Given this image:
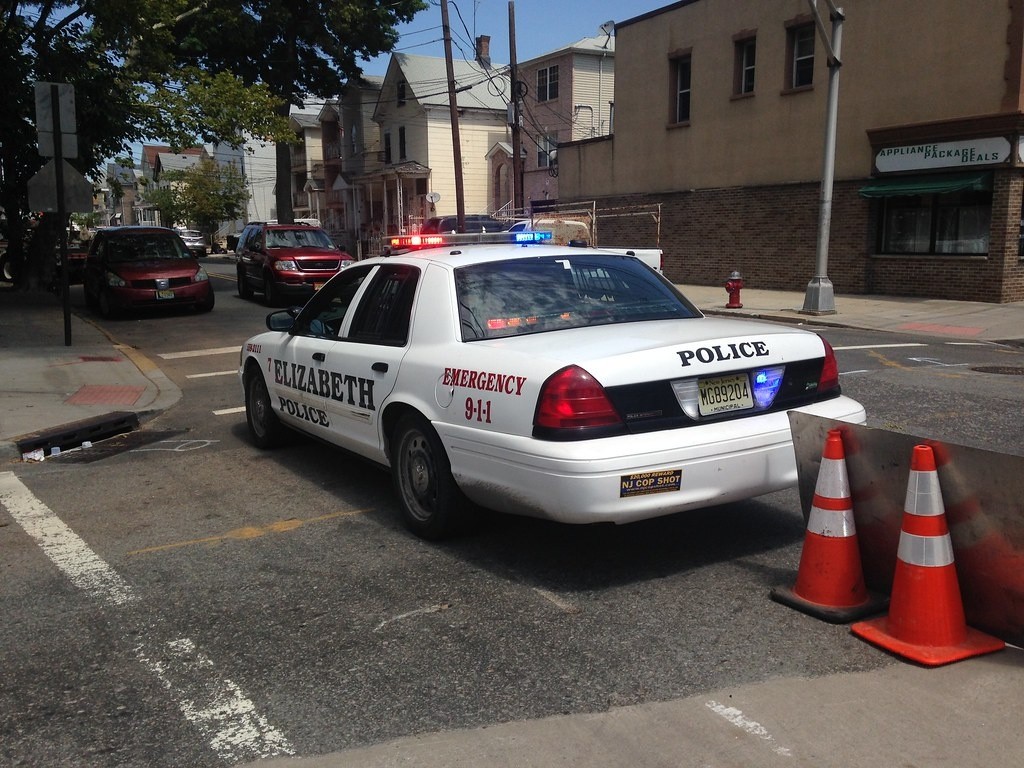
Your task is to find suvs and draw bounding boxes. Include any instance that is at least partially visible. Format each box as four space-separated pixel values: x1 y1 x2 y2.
419 214 511 234
236 221 355 304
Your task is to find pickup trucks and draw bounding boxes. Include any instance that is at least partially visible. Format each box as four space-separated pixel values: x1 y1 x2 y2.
506 200 663 278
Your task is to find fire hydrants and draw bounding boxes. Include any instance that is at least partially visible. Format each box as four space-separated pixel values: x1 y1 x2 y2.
724 270 745 310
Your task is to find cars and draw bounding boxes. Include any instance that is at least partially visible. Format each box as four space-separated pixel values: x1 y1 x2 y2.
235 223 869 543
0 222 215 321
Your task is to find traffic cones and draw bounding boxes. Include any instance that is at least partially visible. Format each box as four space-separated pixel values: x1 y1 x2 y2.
926 440 1023 637
818 423 899 576
768 428 892 623
852 445 1008 668
